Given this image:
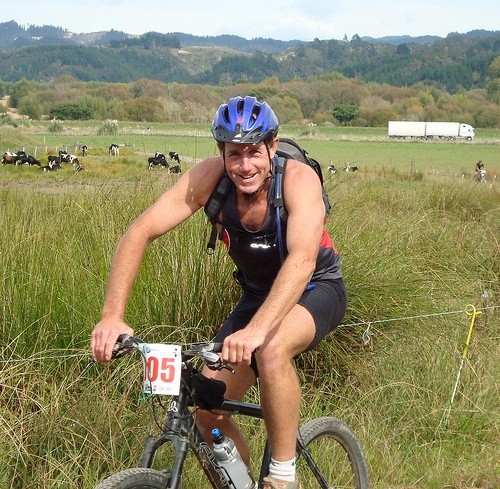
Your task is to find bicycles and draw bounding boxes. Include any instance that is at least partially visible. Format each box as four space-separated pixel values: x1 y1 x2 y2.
473 169 486 183
90 329 371 489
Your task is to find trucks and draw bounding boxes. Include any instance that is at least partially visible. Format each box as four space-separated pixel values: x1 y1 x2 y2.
387 120 476 142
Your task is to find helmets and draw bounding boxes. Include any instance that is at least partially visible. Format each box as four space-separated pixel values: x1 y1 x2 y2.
210 95 280 145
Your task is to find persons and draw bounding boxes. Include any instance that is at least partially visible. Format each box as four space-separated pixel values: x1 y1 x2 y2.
476 160 484 177
91 96 348 489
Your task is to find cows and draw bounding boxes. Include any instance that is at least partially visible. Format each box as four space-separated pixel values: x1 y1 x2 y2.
80 145 88 156
1 150 81 172
327 160 358 174
108 143 120 156
147 151 181 173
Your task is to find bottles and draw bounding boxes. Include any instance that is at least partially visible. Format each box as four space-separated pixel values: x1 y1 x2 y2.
211 427 258 489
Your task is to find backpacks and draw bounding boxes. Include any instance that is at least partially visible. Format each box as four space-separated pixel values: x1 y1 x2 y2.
203 137 332 292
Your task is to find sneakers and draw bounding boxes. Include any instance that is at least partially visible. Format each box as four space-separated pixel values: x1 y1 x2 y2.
261 474 303 489
